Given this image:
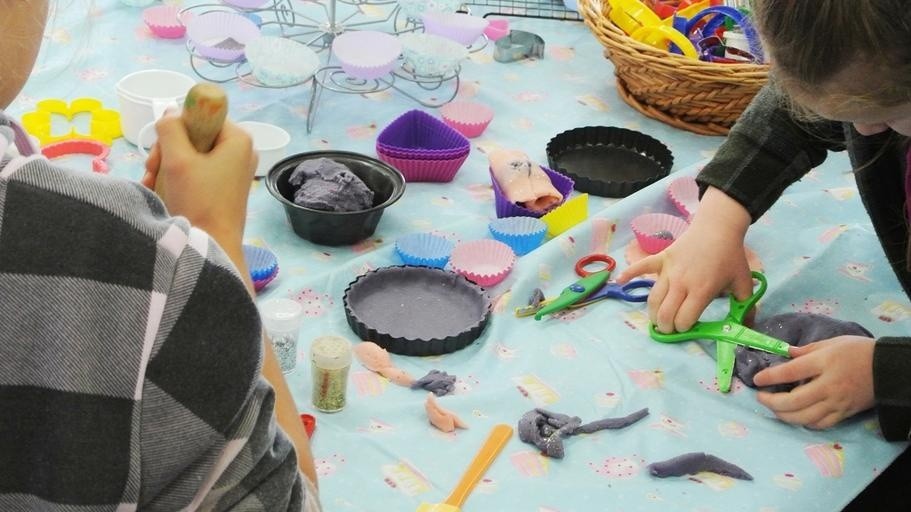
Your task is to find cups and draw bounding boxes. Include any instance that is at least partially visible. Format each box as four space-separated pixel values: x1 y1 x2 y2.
236 122 291 179
313 336 352 417
113 69 196 151
261 297 301 375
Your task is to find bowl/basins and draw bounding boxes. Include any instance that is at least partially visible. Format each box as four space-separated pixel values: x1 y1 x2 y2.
267 149 403 246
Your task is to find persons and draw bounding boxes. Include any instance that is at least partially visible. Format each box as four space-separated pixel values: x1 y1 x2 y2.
1 1 323 512
608 1 910 441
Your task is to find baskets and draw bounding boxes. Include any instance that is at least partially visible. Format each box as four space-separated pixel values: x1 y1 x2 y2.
578 0 773 138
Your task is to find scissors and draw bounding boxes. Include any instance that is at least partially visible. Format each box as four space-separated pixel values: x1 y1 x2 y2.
647 269 793 392
514 254 655 321
